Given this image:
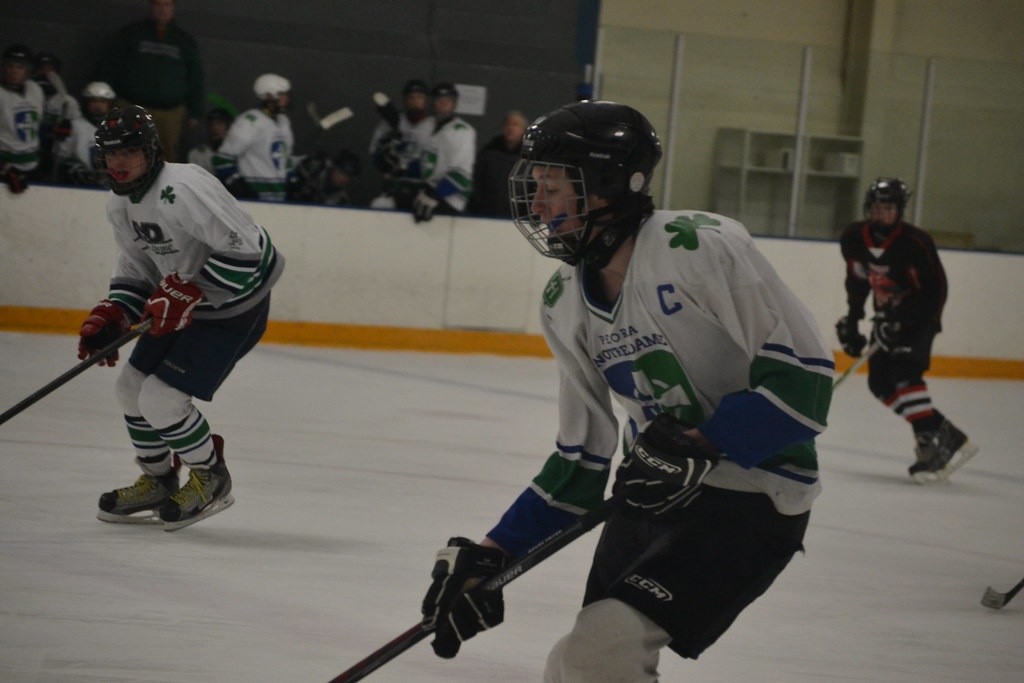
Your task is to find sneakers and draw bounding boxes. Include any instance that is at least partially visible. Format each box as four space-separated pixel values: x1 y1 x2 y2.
159 434 235 532
908 426 978 482
95 451 183 524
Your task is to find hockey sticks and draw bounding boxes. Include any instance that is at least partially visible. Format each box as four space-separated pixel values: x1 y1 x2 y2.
981 578 1024 610
832 340 882 391
0 316 154 428
329 495 621 683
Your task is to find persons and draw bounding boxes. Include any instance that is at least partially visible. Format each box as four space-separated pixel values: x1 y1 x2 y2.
97 0 204 127
425 99 836 683
835 177 980 485
77 105 286 532
0 43 539 225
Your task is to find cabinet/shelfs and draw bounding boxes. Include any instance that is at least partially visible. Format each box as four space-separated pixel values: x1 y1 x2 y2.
710 126 867 240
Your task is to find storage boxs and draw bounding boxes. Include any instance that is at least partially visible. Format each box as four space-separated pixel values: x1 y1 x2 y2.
823 152 859 175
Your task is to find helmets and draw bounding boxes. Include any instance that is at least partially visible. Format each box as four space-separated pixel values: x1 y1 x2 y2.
254 74 291 113
509 100 664 259
863 177 908 230
2 46 30 86
206 108 231 141
433 84 457 116
93 104 164 195
82 81 116 120
402 80 430 114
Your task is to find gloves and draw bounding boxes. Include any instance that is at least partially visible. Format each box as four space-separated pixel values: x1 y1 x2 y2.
78 299 131 366
836 318 866 358
141 271 202 336
612 415 721 523
421 537 504 659
413 187 436 223
2 166 30 194
